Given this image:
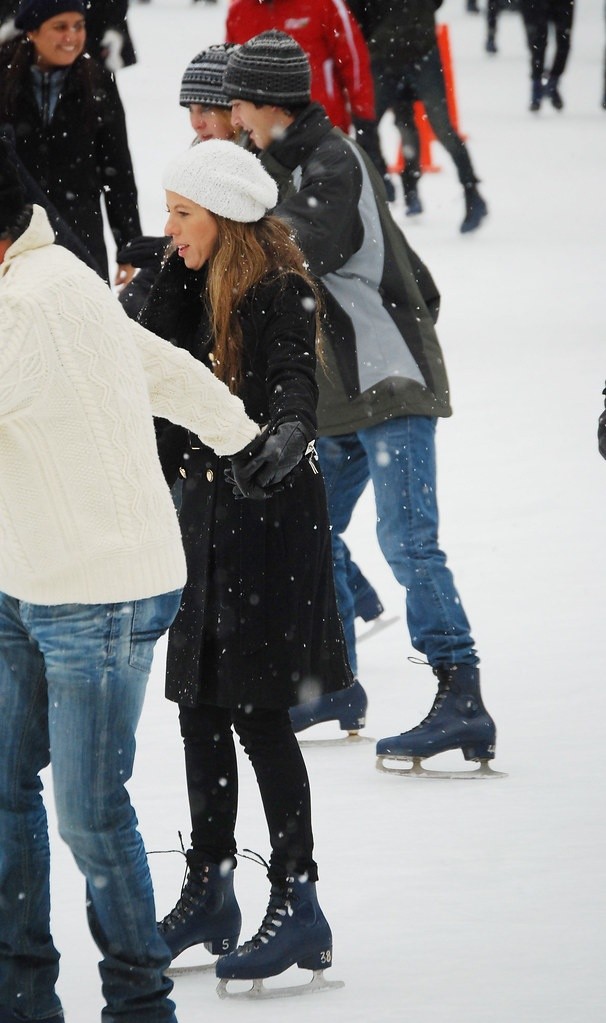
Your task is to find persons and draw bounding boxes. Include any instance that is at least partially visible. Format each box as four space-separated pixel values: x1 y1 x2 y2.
0 0 143 293
132 137 345 1003
115 30 509 780
345 0 575 234
225 0 377 136
0 137 295 1023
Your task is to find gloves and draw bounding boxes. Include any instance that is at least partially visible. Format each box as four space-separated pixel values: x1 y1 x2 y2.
223 422 310 499
118 236 171 268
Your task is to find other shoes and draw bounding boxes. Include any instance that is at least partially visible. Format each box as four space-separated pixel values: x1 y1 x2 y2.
529 84 545 110
485 40 498 53
545 81 563 109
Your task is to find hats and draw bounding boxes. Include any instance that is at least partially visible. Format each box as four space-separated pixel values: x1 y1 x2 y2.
179 41 237 110
221 30 312 103
15 0 91 30
164 138 277 222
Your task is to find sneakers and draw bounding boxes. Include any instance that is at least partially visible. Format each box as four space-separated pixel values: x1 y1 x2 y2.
141 831 242 960
286 679 368 742
214 848 346 999
458 184 489 233
376 656 510 781
348 571 400 642
400 172 423 216
